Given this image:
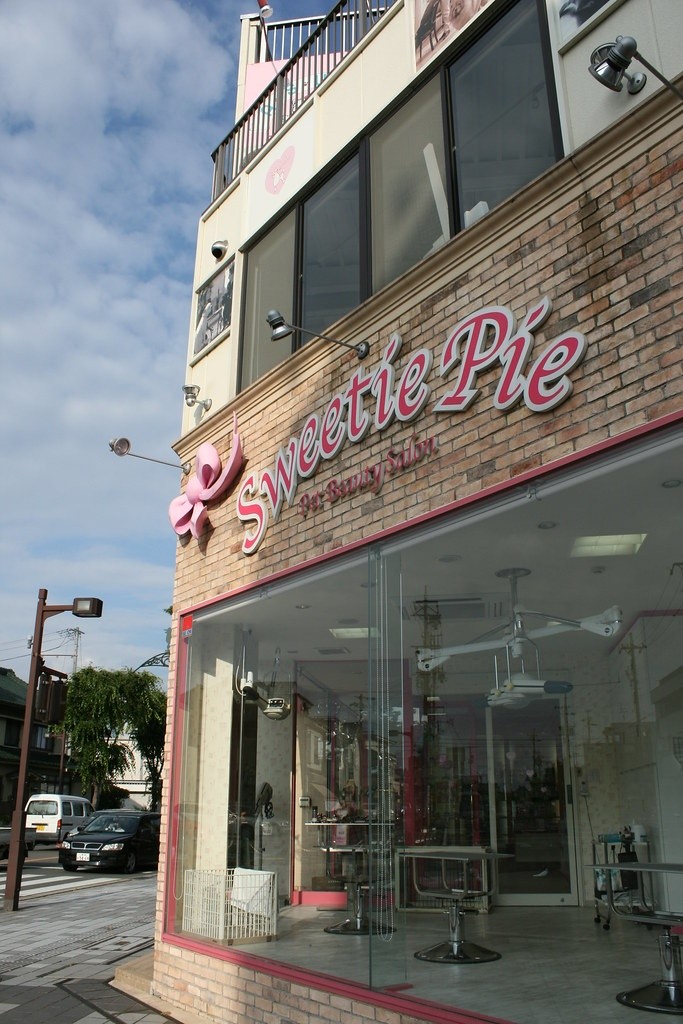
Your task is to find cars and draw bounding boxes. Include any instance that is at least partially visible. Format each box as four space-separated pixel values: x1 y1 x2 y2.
59 808 161 874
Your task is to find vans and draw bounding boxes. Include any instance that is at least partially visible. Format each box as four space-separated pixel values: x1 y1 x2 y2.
24 794 97 851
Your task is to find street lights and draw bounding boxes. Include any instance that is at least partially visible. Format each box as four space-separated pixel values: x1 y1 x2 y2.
3 588 103 912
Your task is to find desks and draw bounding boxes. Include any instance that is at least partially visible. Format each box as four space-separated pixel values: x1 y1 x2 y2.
313 844 399 936
582 861 683 1016
397 849 517 965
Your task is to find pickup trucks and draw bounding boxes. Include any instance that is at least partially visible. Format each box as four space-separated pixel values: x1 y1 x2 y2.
0 826 38 866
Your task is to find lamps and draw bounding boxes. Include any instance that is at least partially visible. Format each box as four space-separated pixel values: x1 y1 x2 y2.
266 310 369 360
108 437 191 475
588 34 683 101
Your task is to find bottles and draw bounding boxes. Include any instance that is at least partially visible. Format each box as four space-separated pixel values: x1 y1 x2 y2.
312 806 318 823
